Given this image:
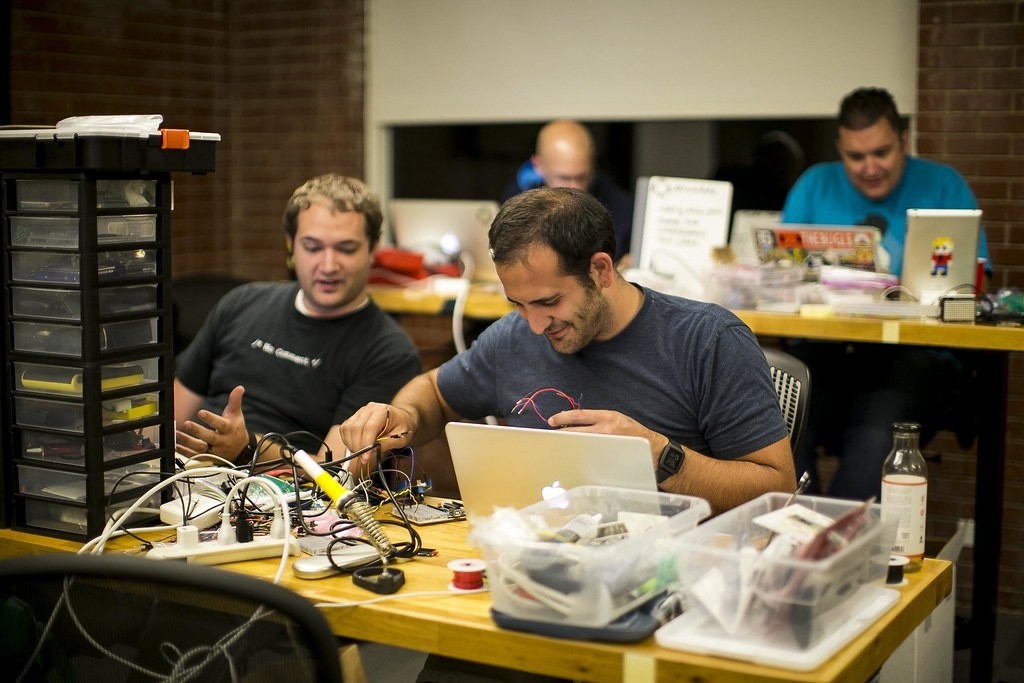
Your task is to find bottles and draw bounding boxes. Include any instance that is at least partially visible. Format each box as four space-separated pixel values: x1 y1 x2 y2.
878 423 928 572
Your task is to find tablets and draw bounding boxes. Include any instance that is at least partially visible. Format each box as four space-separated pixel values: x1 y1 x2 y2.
898 207 984 301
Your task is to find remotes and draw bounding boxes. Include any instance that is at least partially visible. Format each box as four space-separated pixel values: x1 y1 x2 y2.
292 543 381 581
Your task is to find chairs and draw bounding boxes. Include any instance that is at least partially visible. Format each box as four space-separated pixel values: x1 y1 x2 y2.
173 271 253 356
0 552 347 683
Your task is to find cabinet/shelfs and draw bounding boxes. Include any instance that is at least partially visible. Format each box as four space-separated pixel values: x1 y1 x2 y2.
0 174 175 544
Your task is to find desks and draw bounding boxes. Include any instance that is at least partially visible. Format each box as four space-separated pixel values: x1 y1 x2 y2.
369 268 1024 683
0 451 956 683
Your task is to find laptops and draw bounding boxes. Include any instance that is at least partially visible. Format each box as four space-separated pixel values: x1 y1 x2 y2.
389 198 506 284
442 422 659 531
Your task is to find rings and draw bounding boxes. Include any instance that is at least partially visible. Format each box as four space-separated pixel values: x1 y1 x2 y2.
202 442 212 454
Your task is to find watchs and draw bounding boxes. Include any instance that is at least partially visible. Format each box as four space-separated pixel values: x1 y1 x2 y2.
653 437 686 484
226 429 257 469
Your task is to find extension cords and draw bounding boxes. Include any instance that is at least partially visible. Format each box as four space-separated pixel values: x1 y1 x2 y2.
147 533 294 566
161 485 226 531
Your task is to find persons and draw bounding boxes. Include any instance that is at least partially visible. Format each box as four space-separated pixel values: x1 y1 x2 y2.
434 122 637 365
340 186 797 682
1 175 423 683
780 88 992 508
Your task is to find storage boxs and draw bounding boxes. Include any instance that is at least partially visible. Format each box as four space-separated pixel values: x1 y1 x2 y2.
670 491 900 654
0 124 222 178
469 484 711 645
